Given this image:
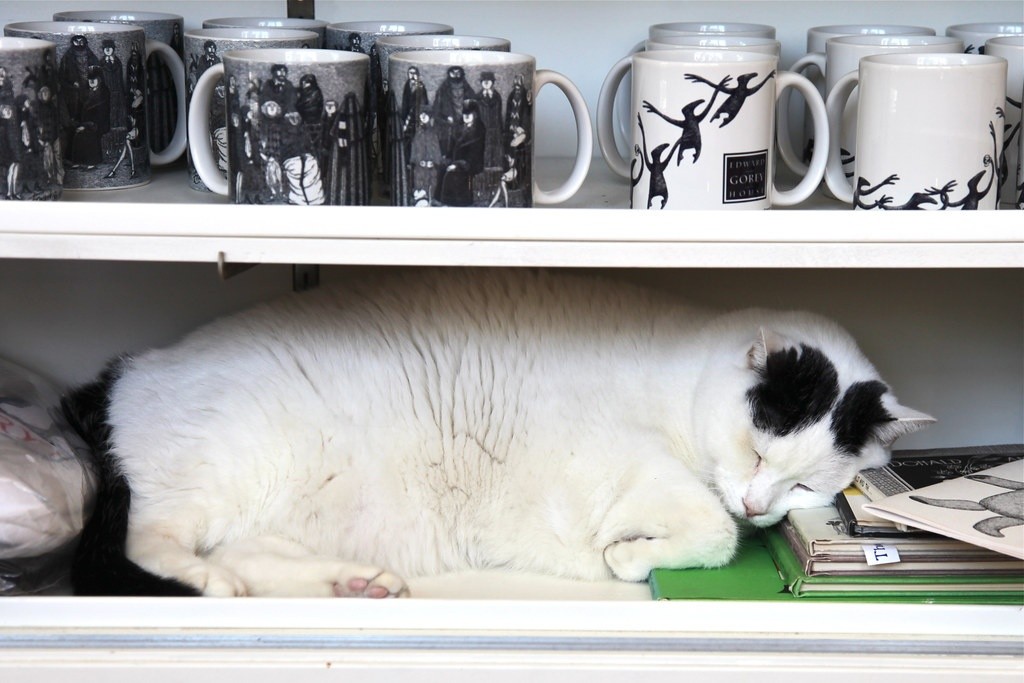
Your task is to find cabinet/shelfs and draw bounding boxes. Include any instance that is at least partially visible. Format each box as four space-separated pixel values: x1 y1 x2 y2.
0 172 1024 683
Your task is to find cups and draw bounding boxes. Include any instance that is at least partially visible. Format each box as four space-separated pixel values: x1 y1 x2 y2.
596 21 1024 212
0 9 594 208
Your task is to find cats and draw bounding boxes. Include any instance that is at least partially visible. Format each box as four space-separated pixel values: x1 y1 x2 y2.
0 266 940 599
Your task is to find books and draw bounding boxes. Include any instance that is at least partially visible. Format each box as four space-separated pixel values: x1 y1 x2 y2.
763 444 1023 601
